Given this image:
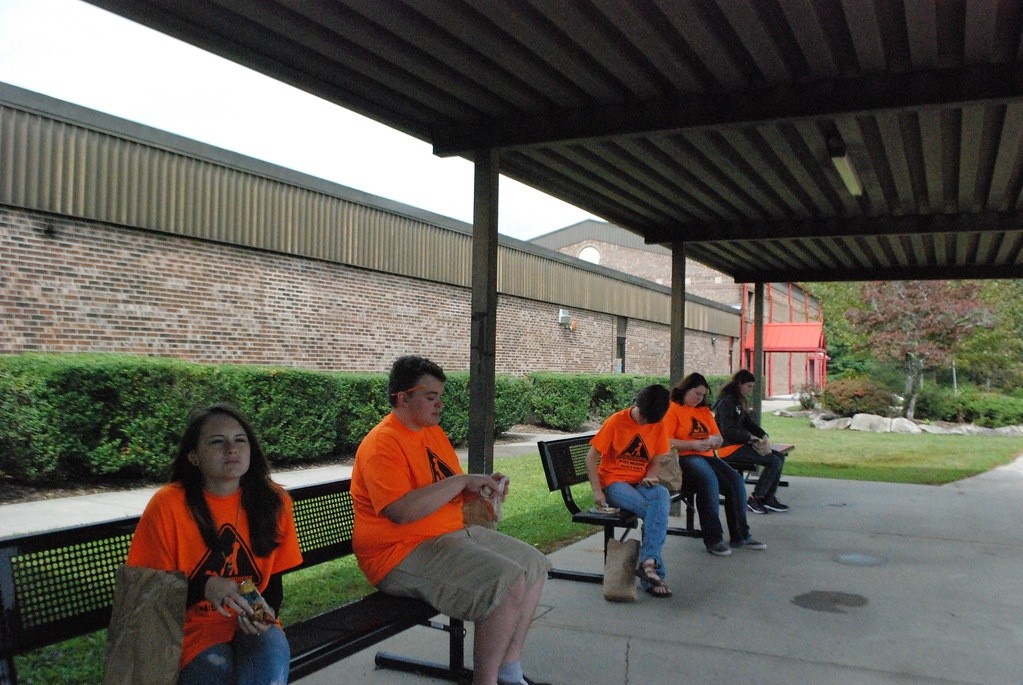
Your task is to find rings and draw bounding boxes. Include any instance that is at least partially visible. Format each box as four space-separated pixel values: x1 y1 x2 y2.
222 595 230 603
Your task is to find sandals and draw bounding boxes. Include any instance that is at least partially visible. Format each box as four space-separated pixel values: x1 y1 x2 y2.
635 563 661 586
640 579 672 597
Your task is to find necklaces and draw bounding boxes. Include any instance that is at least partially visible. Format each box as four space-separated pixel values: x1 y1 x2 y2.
200 485 244 573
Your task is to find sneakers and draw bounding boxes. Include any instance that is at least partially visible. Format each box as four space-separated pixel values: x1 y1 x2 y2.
707 541 731 555
747 496 768 513
730 537 766 549
763 499 789 511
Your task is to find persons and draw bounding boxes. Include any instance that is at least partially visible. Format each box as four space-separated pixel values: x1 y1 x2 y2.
665 372 767 556
584 384 673 597
712 369 790 514
124 403 304 685
349 354 553 685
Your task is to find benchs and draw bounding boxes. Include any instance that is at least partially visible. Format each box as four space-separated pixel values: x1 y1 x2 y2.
0 478 551 685
538 435 796 584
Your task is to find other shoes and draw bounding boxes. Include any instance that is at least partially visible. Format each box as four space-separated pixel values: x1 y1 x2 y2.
499 675 549 685
